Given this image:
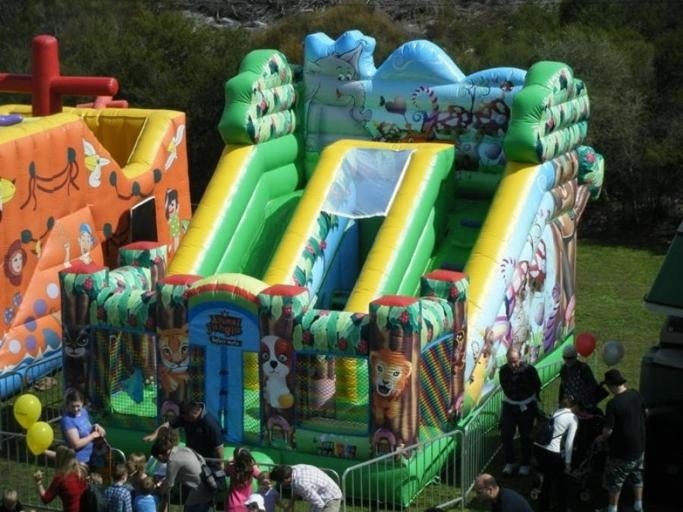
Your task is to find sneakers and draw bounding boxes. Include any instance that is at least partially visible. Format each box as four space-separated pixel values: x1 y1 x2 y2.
595 506 608 512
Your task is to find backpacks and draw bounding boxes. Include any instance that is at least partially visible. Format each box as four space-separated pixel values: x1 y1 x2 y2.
528 414 554 446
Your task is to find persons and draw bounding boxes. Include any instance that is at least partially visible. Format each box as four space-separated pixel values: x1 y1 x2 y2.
142 401 342 512
476 345 652 512
33 392 157 511
1 485 24 511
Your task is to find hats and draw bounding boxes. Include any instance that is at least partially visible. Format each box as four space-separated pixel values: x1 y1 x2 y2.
599 369 627 387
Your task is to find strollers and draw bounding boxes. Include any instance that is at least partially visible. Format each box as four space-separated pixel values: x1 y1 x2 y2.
518 406 593 504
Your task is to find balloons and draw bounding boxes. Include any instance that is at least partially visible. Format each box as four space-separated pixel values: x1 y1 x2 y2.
603 340 624 365
27 421 54 455
576 333 595 357
13 394 42 429
